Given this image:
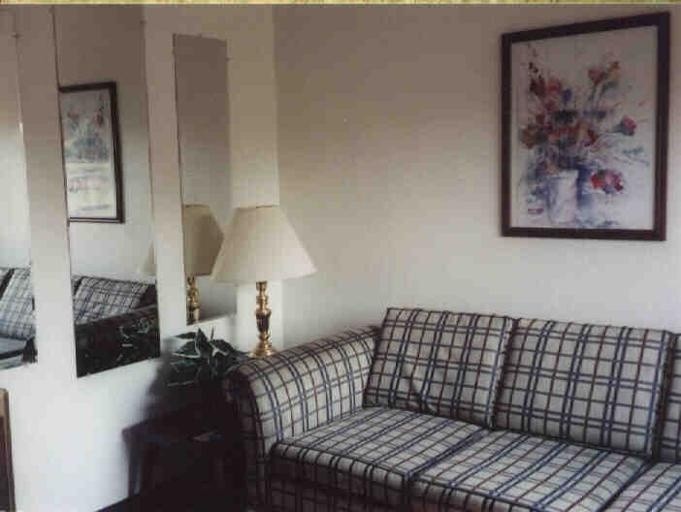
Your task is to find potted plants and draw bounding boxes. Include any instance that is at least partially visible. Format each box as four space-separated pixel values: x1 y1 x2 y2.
168 327 247 418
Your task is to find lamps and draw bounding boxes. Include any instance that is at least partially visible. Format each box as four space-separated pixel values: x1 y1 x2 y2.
135 198 219 327
212 205 315 357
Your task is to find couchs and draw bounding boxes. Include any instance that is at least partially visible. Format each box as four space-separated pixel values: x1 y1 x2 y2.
0 267 158 378
224 307 679 512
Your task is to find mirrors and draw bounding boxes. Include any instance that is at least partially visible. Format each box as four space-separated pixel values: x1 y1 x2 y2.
52 5 163 377
172 32 238 324
0 10 39 374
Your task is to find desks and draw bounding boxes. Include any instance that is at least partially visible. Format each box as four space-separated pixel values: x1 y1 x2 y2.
120 405 243 511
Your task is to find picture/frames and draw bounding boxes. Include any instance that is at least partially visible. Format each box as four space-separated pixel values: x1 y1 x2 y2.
498 12 671 242
57 81 125 226
